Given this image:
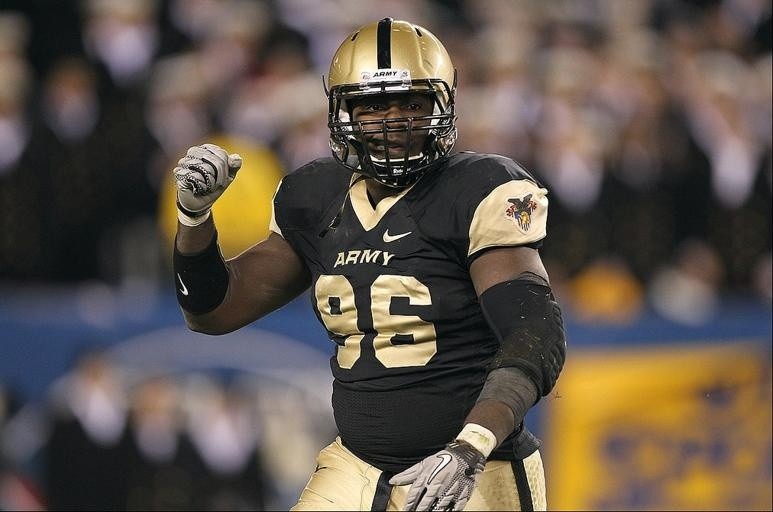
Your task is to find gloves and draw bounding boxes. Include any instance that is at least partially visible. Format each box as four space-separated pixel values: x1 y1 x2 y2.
388 423 497 512
172 143 242 227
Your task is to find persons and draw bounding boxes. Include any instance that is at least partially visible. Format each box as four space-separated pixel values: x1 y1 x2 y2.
171 16 568 511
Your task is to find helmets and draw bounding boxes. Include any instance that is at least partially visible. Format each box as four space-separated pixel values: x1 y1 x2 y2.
321 18 457 190
